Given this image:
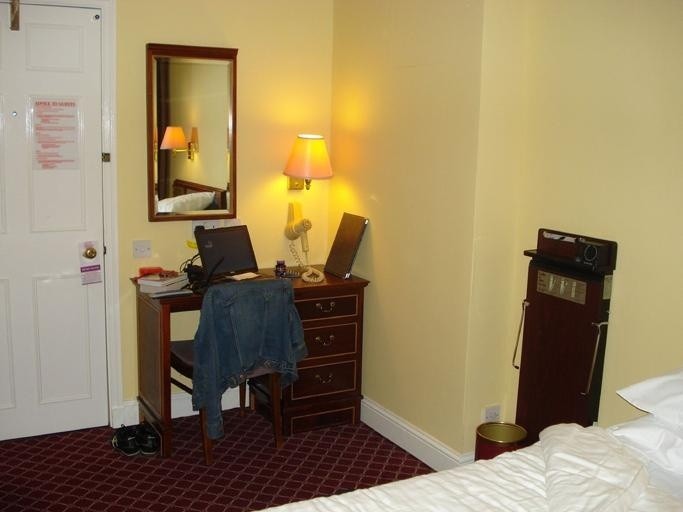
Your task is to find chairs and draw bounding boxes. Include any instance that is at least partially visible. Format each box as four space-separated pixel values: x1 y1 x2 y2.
171 279 291 465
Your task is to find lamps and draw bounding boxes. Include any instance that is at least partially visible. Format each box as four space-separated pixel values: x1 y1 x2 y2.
284 134 333 191
160 127 199 161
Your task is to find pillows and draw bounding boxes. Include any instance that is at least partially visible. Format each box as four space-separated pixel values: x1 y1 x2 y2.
606 414 683 475
607 371 682 424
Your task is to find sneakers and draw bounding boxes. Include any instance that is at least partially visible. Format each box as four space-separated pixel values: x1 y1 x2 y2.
128 425 158 454
112 424 140 456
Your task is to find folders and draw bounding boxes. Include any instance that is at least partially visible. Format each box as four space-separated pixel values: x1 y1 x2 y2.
324 212 368 281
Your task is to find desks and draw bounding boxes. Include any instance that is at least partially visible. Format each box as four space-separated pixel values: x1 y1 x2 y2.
129 264 369 456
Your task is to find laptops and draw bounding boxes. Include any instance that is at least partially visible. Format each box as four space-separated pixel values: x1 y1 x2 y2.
191 225 258 282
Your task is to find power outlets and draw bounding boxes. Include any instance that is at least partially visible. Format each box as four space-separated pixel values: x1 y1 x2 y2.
482 404 501 422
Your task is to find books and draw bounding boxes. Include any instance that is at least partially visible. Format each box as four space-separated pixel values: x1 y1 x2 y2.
137 273 190 293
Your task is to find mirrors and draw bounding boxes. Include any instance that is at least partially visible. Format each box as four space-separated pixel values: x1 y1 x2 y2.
143 44 237 221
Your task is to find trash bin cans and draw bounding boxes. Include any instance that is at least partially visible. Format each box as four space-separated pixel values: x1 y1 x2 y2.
474 423 527 463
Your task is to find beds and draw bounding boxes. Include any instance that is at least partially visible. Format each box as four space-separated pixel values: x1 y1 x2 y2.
256 424 683 511
